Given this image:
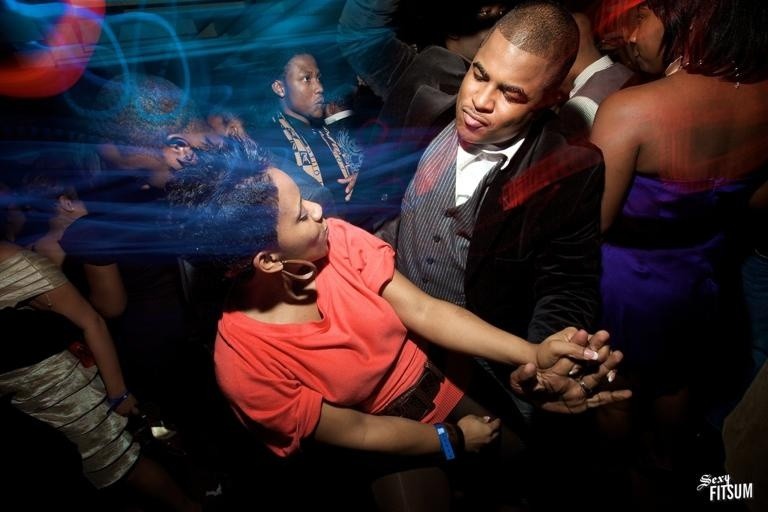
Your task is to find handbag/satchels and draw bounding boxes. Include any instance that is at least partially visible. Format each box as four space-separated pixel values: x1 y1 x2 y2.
132 398 192 470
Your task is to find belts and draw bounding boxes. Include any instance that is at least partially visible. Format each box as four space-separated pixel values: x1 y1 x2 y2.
377 343 449 420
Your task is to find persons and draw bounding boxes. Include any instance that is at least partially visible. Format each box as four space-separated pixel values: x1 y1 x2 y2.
0 0 767 512
